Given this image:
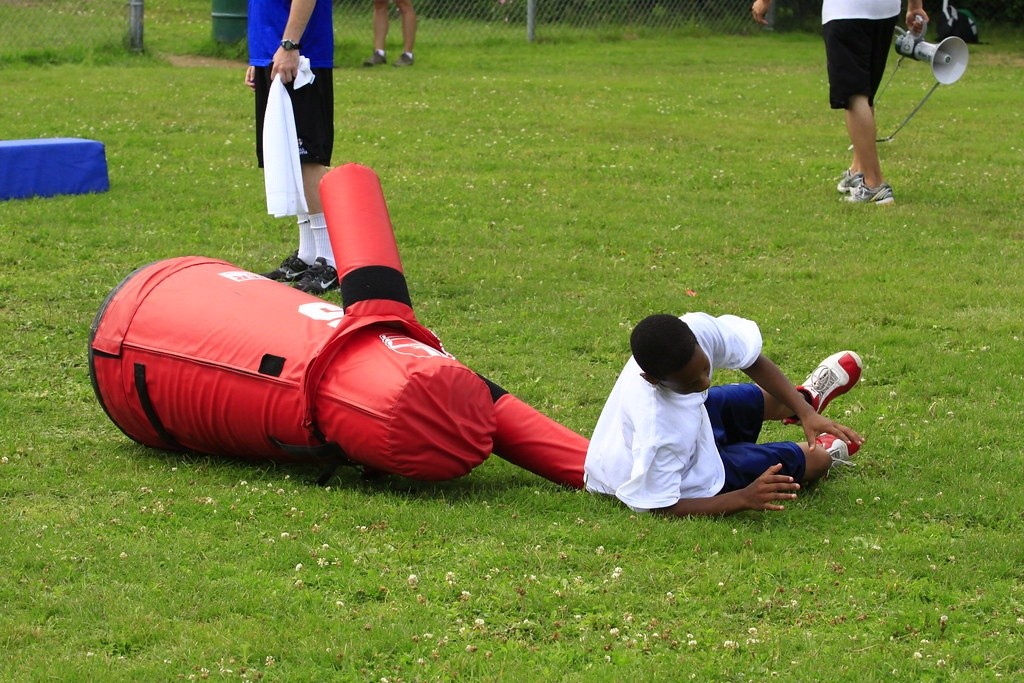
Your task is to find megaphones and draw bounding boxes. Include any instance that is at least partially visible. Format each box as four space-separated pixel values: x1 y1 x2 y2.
894 14 970 86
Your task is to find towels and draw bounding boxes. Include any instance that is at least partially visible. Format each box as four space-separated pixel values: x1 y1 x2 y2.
261 55 316 218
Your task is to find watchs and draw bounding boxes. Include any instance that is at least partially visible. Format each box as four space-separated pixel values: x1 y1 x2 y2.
279 39 302 52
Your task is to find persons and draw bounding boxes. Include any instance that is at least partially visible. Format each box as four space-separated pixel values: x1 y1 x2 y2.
361 0 418 67
580 310 864 520
751 0 930 206
243 0 341 298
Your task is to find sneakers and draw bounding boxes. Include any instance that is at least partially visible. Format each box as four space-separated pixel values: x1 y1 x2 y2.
363 50 387 67
259 248 312 282
835 166 865 195
392 53 413 66
779 350 863 429
292 256 341 296
811 431 860 477
838 176 894 205
